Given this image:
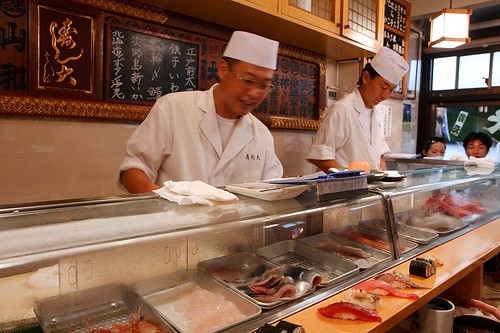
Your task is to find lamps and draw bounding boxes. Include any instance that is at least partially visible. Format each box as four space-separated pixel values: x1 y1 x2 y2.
428 0 472 48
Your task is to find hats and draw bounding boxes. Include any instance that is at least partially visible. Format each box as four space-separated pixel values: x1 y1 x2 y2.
222 30 279 70
371 47 410 85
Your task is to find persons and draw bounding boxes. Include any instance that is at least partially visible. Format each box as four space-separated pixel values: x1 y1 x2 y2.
423 137 446 158
463 132 493 160
306 46 410 174
117 29 283 194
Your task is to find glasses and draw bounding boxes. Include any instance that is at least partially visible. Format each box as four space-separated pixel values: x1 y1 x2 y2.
226 65 274 93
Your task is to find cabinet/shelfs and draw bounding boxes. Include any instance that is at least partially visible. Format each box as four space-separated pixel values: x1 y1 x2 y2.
137 0 385 61
